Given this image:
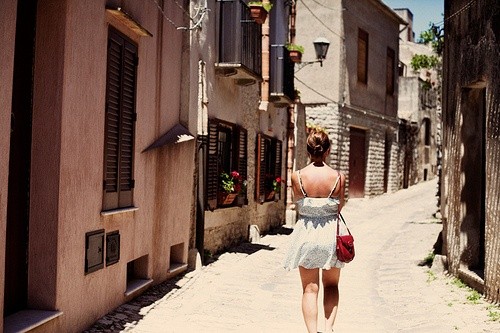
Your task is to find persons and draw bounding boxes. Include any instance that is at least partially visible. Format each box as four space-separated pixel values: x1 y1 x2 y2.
291 131 346 333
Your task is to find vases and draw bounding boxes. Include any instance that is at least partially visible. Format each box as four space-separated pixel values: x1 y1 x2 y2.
218 191 238 204
265 190 275 199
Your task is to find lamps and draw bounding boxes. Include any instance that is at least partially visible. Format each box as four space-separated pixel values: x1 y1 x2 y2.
294 38 330 73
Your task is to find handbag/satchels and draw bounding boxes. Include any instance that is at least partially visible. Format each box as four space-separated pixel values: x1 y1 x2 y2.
335 212 355 263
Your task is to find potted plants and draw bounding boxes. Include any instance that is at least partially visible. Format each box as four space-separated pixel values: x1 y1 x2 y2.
248 2 273 24
285 42 303 64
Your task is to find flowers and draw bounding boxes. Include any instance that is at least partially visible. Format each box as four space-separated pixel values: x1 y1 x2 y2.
221 171 240 192
273 177 280 190
